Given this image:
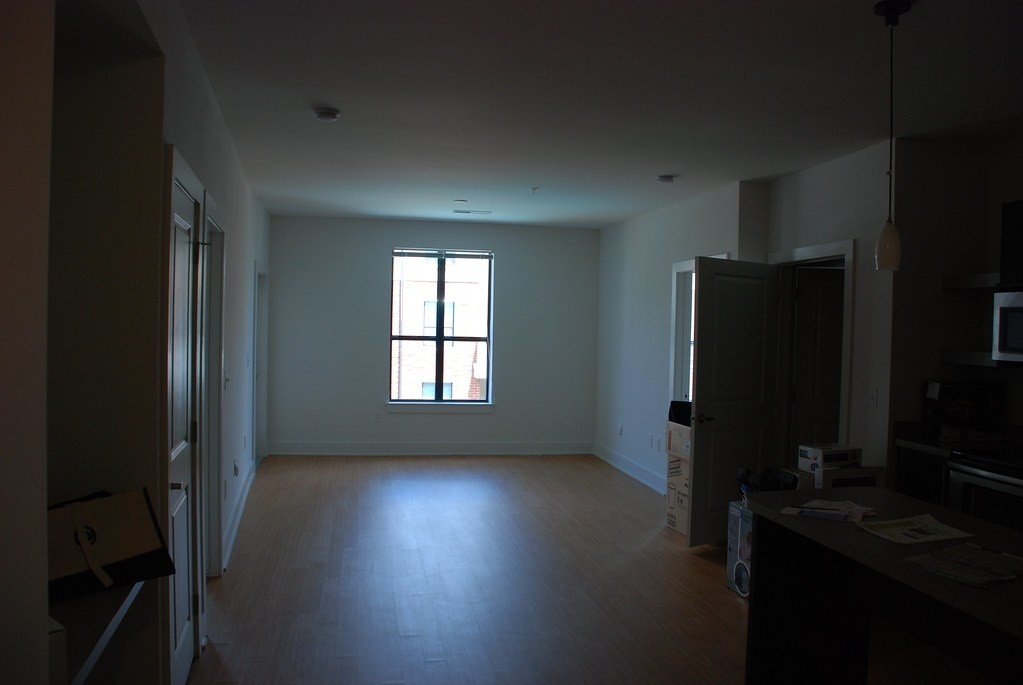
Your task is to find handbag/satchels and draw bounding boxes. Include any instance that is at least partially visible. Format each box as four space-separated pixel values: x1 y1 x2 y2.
49 483 176 601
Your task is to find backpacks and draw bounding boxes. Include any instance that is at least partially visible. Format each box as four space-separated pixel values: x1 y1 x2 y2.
730 461 799 499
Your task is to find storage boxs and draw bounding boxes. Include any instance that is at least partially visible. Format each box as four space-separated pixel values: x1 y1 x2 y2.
725 500 754 600
781 444 885 489
665 421 692 536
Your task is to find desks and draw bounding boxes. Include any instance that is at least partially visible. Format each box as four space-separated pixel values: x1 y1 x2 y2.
743 487 1023 685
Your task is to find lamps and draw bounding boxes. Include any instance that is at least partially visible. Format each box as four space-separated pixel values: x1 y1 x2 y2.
874 0 916 272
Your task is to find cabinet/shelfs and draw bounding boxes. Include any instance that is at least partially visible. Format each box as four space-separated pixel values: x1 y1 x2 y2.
897 448 945 505
